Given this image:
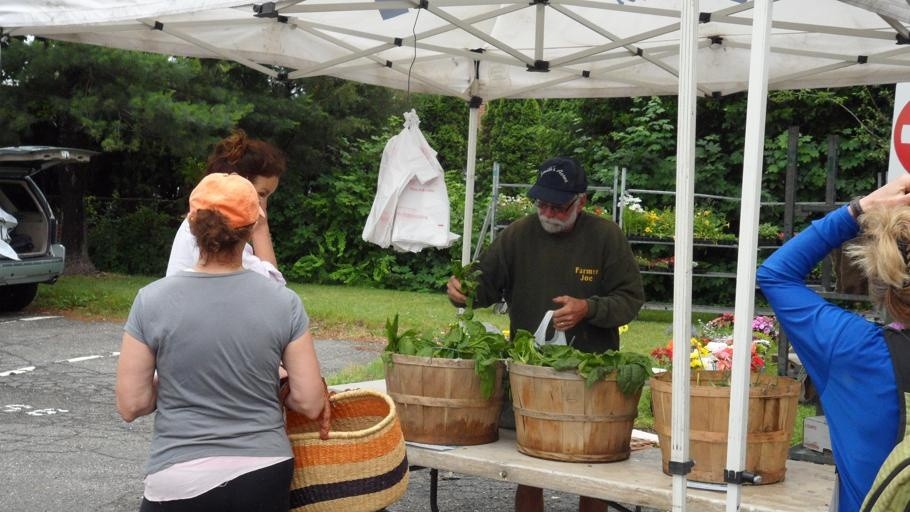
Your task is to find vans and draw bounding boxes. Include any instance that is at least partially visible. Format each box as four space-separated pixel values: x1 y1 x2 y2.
0 143 107 315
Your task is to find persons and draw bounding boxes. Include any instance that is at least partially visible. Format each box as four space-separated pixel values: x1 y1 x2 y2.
756 173 910 511
114 174 328 511
164 131 287 285
444 155 650 511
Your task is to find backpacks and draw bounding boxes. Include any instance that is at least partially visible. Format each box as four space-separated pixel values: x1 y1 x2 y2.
861 318 908 512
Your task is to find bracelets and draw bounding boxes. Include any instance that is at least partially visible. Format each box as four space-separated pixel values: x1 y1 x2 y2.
848 195 866 217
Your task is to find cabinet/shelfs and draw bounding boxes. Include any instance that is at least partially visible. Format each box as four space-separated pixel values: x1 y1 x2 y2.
469 161 620 317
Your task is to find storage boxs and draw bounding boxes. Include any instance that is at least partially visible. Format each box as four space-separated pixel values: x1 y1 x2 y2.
801 414 834 454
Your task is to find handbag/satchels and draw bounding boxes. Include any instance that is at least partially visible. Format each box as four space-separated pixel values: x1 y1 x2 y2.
268 373 409 512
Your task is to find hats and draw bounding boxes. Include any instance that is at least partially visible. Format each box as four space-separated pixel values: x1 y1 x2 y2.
186 172 265 223
527 154 587 204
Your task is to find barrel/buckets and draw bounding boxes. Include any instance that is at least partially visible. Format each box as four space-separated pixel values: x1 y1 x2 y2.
382 352 504 446
505 360 643 463
649 371 803 486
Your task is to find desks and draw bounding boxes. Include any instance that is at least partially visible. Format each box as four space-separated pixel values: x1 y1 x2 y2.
326 379 840 511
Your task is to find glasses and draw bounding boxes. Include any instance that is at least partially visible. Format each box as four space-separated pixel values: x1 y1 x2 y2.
537 196 578 212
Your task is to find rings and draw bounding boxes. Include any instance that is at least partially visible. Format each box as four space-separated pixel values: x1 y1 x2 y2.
560 321 565 328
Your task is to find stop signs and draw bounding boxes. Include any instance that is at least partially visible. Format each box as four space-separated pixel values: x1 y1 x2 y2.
896 99 910 172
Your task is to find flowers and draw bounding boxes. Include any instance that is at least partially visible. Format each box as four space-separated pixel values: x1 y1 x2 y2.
648 337 767 392
492 190 617 248
699 312 787 347
